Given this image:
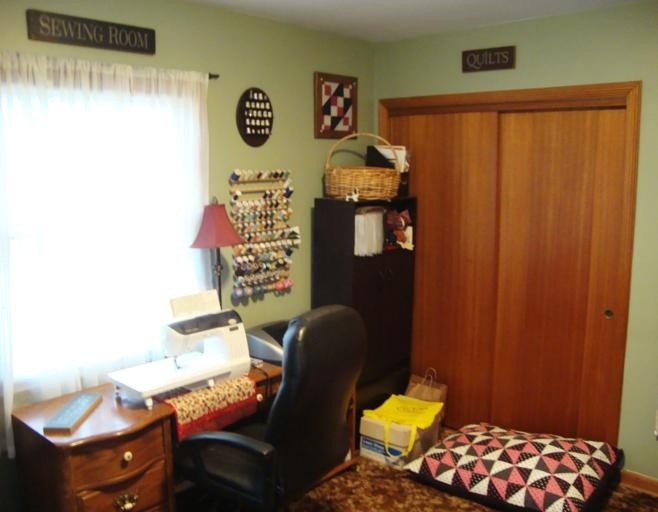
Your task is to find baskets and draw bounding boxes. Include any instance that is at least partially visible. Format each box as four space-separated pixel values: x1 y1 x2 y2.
324 134 400 201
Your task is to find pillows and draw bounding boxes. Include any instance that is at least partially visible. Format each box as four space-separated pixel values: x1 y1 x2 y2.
400 418 627 512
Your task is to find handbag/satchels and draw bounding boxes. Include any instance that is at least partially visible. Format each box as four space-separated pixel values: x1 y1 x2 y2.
363 394 444 431
406 369 447 420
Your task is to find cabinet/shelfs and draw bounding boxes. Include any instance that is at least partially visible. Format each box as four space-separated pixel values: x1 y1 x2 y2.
310 196 416 450
376 80 643 449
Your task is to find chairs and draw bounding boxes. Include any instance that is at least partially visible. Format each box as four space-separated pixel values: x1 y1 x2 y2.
174 303 369 512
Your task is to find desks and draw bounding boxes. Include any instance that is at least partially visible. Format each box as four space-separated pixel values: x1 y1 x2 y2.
8 358 360 512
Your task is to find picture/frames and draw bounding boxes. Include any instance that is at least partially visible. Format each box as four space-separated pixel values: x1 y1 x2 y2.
312 71 358 140
